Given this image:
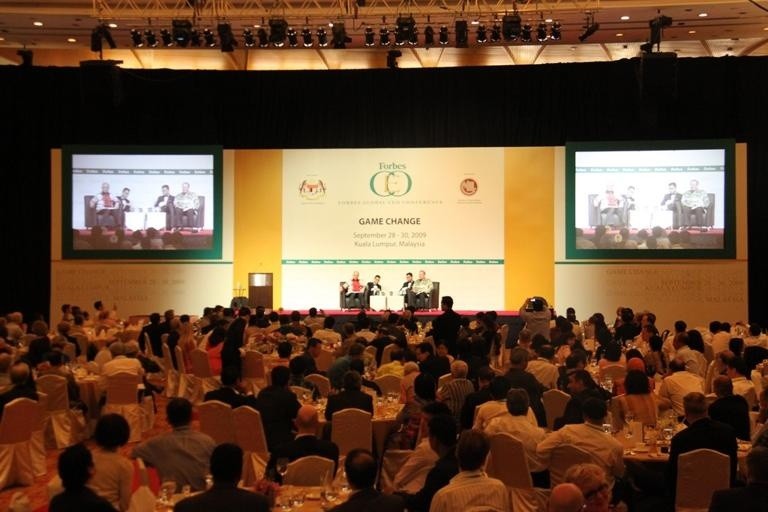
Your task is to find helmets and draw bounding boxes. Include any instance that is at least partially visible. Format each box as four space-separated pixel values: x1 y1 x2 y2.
305 493 321 501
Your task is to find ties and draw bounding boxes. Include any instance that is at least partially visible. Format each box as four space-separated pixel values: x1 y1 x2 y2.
60 145 224 260
565 143 737 260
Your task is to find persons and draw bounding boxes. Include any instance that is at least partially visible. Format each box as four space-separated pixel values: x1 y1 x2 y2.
314 315 342 349
271 341 291 368
99 342 142 418
53 321 80 364
39 349 88 416
155 184 176 232
669 392 737 488
681 180 710 232
563 464 611 511
204 356 256 411
254 365 301 457
641 313 656 326
173 442 271 511
384 401 452 494
597 342 626 379
576 224 695 250
557 333 575 363
525 344 558 391
658 357 706 434
340 271 365 309
435 340 454 364
392 414 459 512
712 322 732 354
491 347 547 428
60 299 117 341
130 396 217 497
643 335 664 377
556 349 612 400
88 183 121 229
73 225 183 249
138 304 226 375
0 363 38 419
460 311 501 356
0 352 15 394
7 312 25 340
660 182 683 231
330 341 365 387
703 320 720 343
326 448 405 512
519 295 552 347
297 337 328 377
707 446 768 512
552 369 613 430
363 274 381 309
398 272 414 309
471 376 538 431
516 329 535 361
548 307 605 344
251 304 327 334
405 270 433 310
398 361 420 404
534 397 627 511
24 320 51 367
548 483 586 512
640 323 657 356
86 411 135 511
428 431 510 512
124 339 165 400
436 360 476 419
432 295 460 357
593 182 637 229
220 305 250 384
321 369 376 466
415 341 450 383
673 331 707 377
383 373 436 450
743 323 765 345
115 188 132 229
619 368 671 427
727 356 756 412
615 307 642 341
286 357 320 401
48 441 116 512
172 182 201 233
709 375 751 441
341 309 419 363
482 388 550 489
662 320 686 367
262 403 339 487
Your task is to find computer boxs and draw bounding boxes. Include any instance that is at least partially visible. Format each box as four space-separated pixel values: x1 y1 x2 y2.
386 50 402 69
16 48 33 66
648 15 673 46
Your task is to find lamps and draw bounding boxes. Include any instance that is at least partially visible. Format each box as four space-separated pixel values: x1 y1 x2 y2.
200 400 231 446
314 349 336 371
374 374 404 396
98 372 149 442
497 323 508 365
229 406 272 484
174 345 193 397
36 374 89 449
161 343 178 398
243 349 270 396
490 432 532 488
280 456 335 484
541 388 570 427
1 398 43 489
188 348 221 401
304 374 331 394
674 449 731 509
549 444 597 486
331 407 373 451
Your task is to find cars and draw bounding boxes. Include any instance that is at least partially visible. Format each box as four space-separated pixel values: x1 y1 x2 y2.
155 208 161 214
381 292 386 297
137 208 143 213
146 207 152 214
389 292 393 297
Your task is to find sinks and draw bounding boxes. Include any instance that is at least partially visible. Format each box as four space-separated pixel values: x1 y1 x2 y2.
340 282 367 312
404 282 439 311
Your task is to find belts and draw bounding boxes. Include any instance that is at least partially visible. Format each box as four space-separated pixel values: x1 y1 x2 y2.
320 470 333 497
605 377 614 394
319 399 327 413
603 423 611 437
622 425 634 456
663 429 673 453
276 457 289 480
398 291 405 296
302 391 310 405
324 490 337 502
280 495 304 512
590 359 597 369
624 411 634 441
315 397 323 409
387 392 399 410
336 480 354 503
